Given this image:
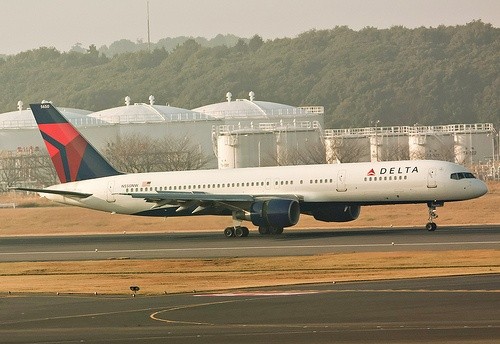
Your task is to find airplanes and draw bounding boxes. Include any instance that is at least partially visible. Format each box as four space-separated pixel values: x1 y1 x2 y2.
6 104 488 238
